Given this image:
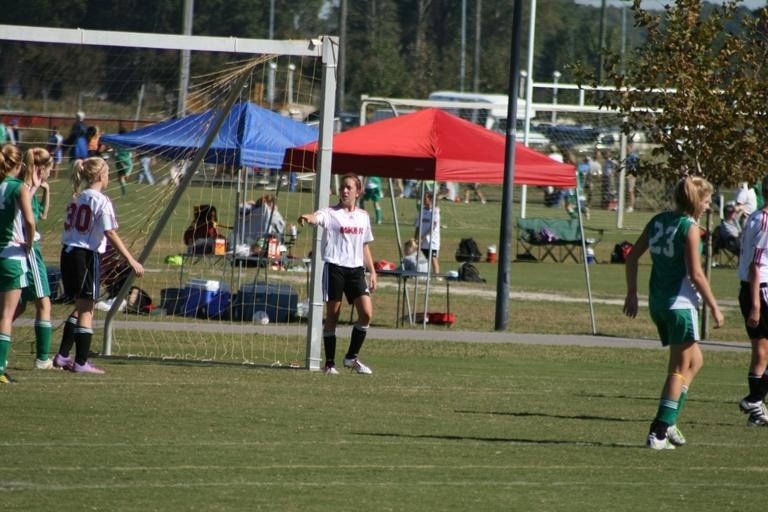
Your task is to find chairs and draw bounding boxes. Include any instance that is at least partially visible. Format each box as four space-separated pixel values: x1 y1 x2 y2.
241 232 281 256
187 240 214 255
714 225 739 269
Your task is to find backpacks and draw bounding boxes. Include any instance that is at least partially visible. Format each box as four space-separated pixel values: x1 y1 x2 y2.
454 237 482 262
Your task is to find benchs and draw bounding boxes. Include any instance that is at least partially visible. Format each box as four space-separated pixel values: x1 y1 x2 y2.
515 217 602 263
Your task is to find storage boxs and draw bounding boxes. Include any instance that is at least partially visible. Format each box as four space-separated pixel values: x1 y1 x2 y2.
232 283 298 323
181 278 231 319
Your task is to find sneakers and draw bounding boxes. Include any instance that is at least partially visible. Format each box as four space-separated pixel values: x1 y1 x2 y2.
665 424 686 445
0 373 16 384
33 358 62 370
53 354 73 371
647 433 676 450
739 399 768 426
343 357 373 374
325 365 340 375
72 362 104 375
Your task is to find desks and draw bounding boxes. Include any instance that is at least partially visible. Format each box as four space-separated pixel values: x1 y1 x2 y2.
232 255 311 301
350 269 457 328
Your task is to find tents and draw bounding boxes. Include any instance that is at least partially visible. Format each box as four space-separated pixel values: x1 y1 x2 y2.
251 111 598 333
93 98 320 322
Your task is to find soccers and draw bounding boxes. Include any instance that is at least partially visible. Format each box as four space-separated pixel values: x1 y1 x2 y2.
253 311 269 326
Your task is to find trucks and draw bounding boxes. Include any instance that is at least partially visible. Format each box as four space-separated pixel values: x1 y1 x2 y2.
426 87 550 151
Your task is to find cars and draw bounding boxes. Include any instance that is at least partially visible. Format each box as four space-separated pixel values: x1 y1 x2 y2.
531 123 661 154
300 109 370 130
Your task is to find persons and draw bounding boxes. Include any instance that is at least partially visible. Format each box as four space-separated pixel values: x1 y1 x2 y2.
2 113 145 384
114 127 190 195
622 175 724 449
737 178 766 428
358 174 486 226
541 142 639 212
415 191 443 282
183 194 294 268
702 172 766 268
297 175 377 374
394 237 418 278
